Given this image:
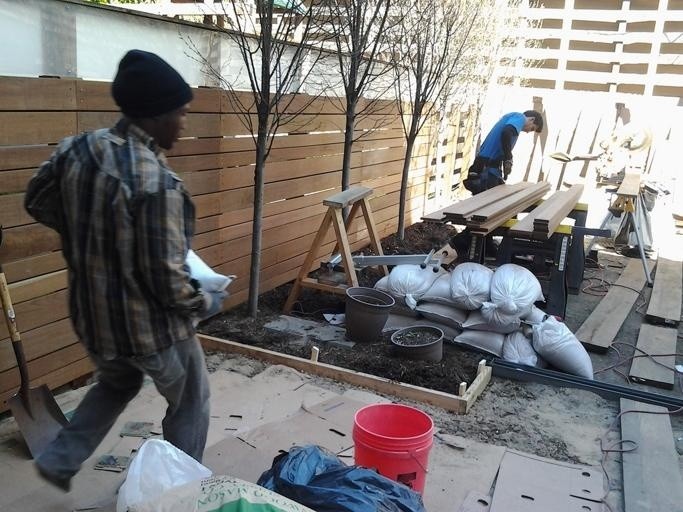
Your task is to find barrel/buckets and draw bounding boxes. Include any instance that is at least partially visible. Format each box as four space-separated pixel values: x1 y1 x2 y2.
351 401 435 496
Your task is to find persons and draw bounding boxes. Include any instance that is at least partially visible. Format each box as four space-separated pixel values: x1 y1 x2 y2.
22 47 228 494
452 110 544 257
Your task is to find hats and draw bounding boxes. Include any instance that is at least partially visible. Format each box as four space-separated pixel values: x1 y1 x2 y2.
110 50 194 121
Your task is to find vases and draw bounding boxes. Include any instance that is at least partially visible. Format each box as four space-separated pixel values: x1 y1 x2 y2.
391 325 444 363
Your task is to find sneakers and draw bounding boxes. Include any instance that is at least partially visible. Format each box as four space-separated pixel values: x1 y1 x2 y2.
35 464 72 491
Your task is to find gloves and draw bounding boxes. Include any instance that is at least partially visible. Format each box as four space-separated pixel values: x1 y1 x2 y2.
200 292 227 320
503 159 512 179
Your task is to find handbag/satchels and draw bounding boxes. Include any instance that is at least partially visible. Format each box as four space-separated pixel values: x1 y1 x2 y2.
463 163 489 193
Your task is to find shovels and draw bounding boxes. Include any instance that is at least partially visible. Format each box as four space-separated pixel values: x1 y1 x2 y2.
0 266 71 461
